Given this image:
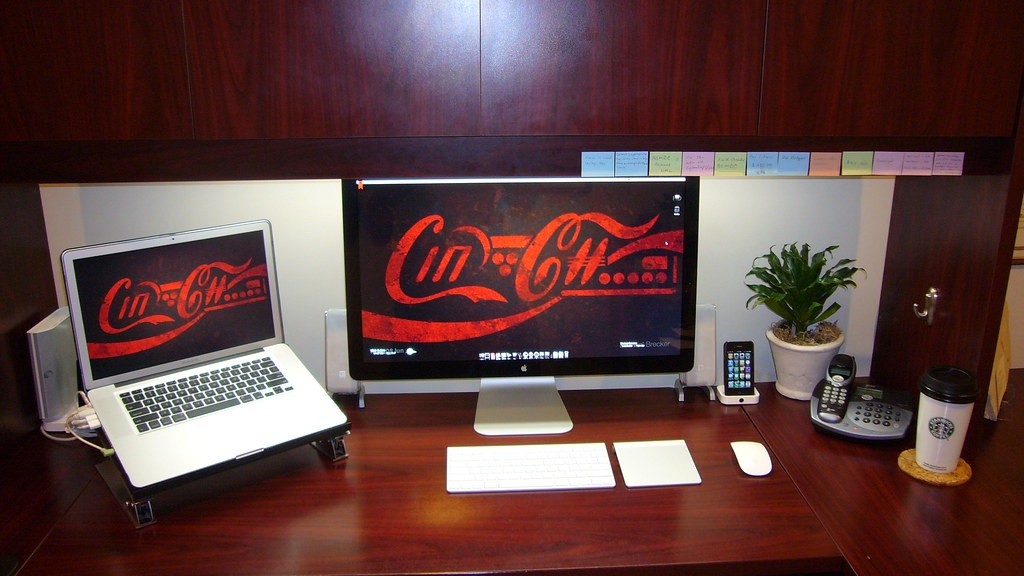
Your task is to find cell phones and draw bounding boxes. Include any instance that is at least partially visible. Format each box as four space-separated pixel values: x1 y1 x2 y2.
724 340 754 396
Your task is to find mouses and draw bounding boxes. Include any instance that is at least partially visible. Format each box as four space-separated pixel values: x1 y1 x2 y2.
730 441 772 476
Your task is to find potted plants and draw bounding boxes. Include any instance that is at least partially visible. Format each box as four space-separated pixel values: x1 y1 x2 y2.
743 242 868 401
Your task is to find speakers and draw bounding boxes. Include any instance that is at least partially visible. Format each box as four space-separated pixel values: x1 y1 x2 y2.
27 307 82 432
675 304 718 402
324 309 366 407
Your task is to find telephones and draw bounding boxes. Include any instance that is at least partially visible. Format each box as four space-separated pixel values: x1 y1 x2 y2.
810 353 913 439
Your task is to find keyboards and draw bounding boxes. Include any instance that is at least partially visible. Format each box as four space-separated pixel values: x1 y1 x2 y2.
446 442 617 494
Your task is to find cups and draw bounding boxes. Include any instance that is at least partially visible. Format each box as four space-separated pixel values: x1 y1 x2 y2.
916 365 978 473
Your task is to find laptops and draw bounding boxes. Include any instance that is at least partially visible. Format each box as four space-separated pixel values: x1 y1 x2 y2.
56 219 351 497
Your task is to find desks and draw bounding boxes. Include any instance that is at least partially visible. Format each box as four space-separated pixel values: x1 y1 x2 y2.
0 368 1024 576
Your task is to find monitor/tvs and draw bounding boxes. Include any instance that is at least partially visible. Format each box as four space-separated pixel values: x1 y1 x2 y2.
340 177 701 435
